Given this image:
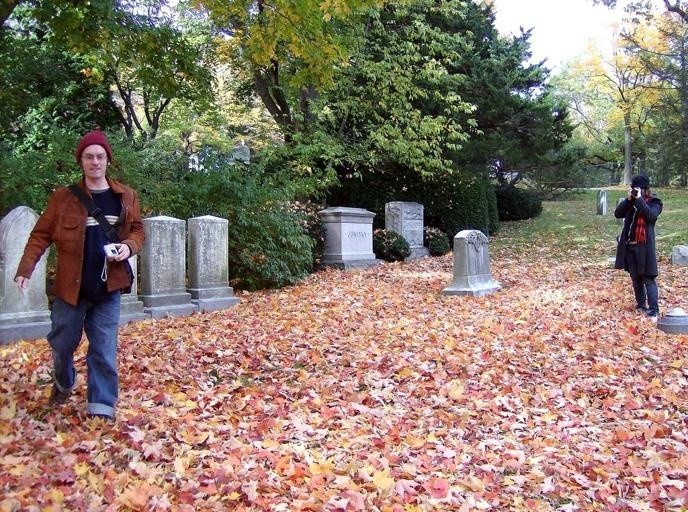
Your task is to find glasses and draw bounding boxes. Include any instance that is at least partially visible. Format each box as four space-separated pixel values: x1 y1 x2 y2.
82 155 108 160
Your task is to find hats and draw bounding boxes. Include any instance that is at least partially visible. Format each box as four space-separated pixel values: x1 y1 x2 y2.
631 176 649 189
76 130 112 166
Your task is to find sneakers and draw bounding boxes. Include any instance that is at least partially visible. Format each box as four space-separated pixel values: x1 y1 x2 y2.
646 308 659 317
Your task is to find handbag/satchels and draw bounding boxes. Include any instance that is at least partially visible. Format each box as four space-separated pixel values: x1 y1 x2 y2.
120 259 135 295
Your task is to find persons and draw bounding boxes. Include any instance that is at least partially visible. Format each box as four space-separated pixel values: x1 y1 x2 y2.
14 129 145 420
615 175 664 316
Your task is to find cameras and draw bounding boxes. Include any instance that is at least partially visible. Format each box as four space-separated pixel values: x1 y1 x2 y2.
631 189 637 196
103 242 120 258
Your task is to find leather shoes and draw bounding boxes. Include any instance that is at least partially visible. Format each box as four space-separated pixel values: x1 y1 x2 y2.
49 382 68 404
